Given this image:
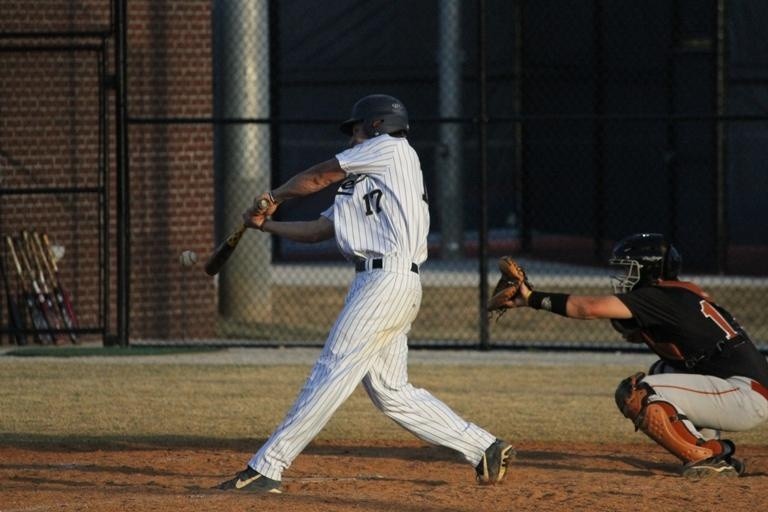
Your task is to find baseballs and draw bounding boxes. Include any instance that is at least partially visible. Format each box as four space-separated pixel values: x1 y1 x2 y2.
180 251 197 267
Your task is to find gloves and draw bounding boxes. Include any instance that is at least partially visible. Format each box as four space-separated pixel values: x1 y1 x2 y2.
243 192 279 232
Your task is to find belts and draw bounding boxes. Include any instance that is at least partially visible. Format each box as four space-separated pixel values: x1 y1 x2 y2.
356 259 418 273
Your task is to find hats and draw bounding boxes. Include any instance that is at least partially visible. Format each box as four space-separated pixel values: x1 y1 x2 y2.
340 94 409 138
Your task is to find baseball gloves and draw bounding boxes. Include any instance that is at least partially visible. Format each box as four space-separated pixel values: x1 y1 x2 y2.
484 255 531 312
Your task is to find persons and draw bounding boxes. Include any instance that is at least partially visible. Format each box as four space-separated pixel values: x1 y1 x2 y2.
487 233 768 479
208 94 513 495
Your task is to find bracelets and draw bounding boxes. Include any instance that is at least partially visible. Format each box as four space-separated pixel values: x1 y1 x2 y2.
527 289 570 318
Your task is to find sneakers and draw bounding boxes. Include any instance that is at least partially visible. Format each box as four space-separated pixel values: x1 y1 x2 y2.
475 440 512 486
684 440 745 481
210 467 282 494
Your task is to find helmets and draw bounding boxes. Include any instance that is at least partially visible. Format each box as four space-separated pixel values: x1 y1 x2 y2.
608 233 680 280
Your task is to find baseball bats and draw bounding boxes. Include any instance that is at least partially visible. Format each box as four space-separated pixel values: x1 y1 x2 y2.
205 200 267 275
2 230 81 346
